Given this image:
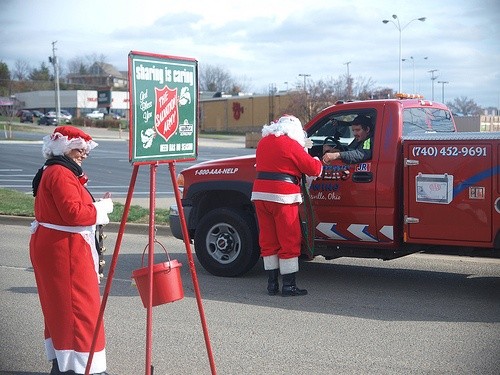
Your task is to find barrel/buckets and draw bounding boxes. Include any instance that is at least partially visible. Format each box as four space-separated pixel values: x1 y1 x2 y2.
130 240 186 308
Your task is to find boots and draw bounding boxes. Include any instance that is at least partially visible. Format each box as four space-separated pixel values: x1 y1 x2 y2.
267 270 279 295
281 273 307 296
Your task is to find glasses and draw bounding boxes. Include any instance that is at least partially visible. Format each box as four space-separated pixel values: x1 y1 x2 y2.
74 149 88 159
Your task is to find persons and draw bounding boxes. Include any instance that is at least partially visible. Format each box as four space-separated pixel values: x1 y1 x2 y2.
323 115 373 165
29 125 113 375
252 115 322 298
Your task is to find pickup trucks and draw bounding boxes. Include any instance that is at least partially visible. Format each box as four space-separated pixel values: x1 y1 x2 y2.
167 91 500 268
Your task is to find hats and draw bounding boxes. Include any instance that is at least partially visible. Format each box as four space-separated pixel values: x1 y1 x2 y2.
260 114 302 143
42 126 98 158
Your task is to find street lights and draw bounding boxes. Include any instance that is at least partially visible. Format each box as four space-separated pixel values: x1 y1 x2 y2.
382 14 427 91
346 61 351 100
48 40 62 128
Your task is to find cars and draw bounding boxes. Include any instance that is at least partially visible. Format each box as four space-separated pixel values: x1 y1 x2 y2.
59 110 72 119
85 110 121 120
37 111 71 125
16 110 35 123
31 111 44 118
